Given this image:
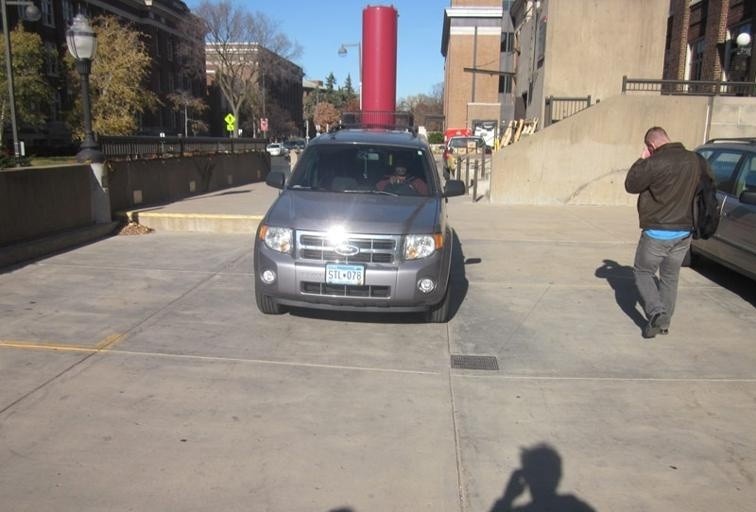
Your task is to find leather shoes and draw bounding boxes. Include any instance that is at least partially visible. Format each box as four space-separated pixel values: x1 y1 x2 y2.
643 312 671 338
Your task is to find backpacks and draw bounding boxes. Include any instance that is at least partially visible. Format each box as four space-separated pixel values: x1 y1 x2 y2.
693 152 720 240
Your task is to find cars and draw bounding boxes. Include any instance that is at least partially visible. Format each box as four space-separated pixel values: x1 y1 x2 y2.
444 133 487 170
266 141 286 158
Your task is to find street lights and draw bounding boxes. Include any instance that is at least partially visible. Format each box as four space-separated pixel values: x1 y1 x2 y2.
63 10 107 164
336 39 363 112
0 0 42 167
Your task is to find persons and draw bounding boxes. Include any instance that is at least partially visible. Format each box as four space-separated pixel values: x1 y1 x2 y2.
484 443 596 512
443 146 456 182
376 159 430 194
624 127 720 338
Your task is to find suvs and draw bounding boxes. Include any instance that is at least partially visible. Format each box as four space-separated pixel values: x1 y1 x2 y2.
686 133 756 284
246 127 466 325
283 136 307 160
